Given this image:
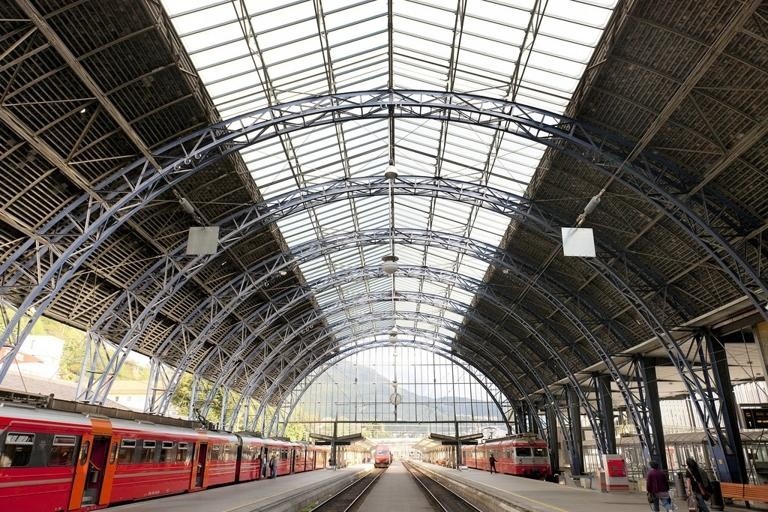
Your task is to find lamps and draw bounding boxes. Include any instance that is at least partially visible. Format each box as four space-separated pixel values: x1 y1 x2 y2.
385 158 398 179
382 253 399 275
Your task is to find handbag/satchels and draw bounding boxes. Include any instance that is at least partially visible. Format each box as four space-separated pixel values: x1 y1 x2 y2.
687 490 699 512
702 493 709 501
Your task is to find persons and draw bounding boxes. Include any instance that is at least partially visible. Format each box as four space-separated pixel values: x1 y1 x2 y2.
684 458 711 512
271 454 278 480
488 453 499 476
645 459 675 512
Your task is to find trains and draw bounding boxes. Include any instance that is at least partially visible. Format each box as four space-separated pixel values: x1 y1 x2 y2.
374 443 393 469
421 432 553 478
1 401 373 512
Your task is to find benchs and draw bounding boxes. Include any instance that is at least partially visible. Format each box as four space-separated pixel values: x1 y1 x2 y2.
719 482 768 509
